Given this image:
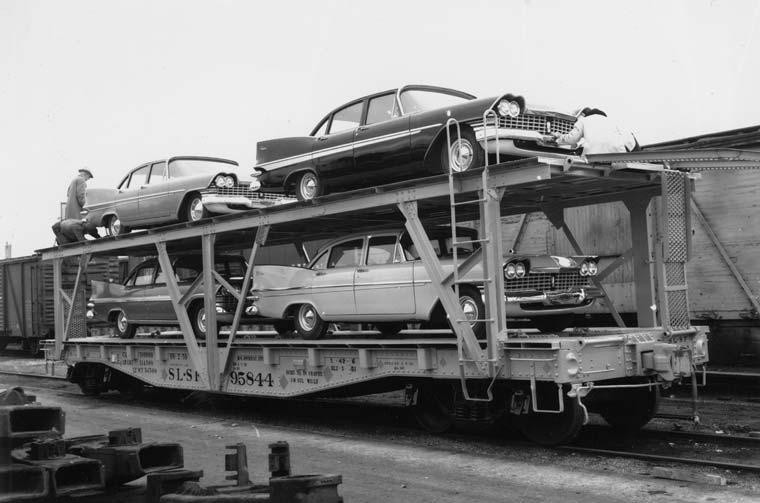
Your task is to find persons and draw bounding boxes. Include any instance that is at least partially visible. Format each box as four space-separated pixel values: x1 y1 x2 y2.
550 105 640 155
51 166 100 245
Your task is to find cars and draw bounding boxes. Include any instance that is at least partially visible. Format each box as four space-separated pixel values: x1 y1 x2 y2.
246 224 608 341
249 82 585 203
86 251 297 339
82 153 299 237
